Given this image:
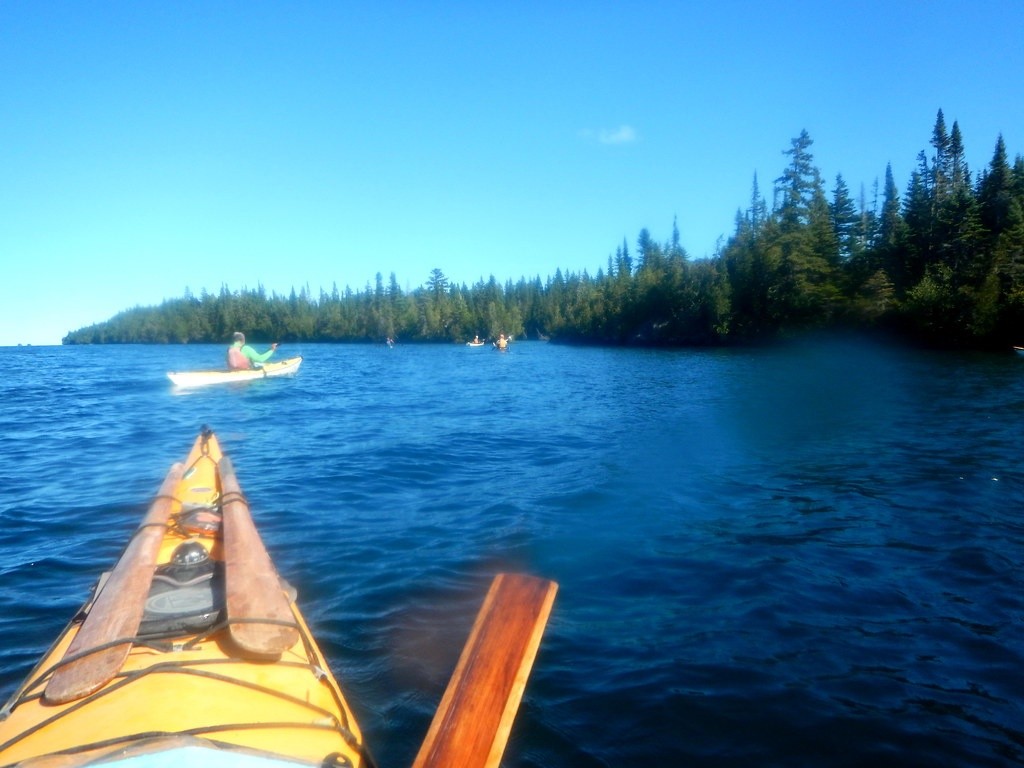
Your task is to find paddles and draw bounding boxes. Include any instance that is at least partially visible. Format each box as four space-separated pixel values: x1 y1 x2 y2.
44 463 185 706
217 456 300 656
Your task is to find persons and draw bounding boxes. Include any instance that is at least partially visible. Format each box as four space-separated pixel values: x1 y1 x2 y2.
474 335 480 344
496 335 507 347
226 331 277 370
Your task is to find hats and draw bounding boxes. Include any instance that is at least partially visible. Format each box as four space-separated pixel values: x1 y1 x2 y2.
499 334 504 338
475 336 478 339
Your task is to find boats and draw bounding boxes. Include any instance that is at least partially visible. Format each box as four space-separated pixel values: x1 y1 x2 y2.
466 341 485 347
165 356 303 388
1 421 378 768
